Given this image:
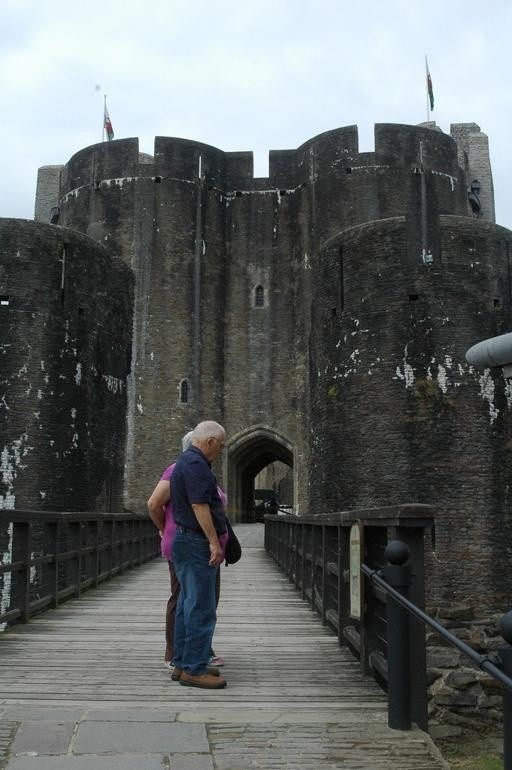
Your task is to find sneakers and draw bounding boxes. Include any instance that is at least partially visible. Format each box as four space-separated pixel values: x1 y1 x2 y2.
165 655 226 689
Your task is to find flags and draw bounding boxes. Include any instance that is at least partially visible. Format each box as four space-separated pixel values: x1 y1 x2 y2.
105 103 115 142
425 60 433 111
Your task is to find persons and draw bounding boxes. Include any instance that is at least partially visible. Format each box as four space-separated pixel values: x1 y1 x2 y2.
148 431 241 671
169 419 231 689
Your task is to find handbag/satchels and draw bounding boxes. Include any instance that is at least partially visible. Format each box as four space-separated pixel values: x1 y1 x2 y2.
225 517 240 566
